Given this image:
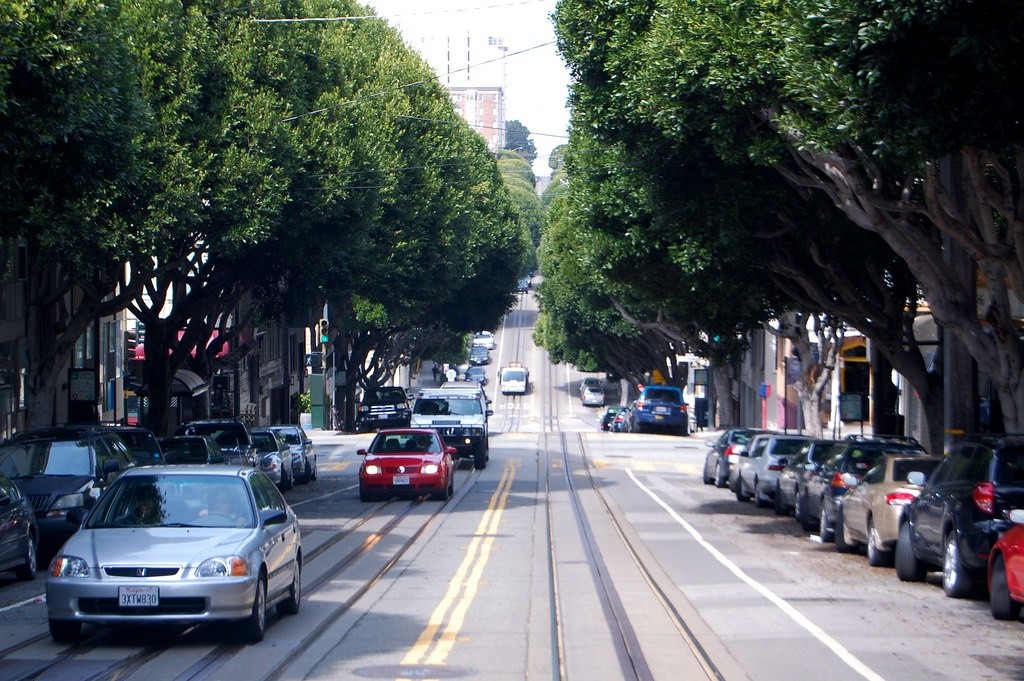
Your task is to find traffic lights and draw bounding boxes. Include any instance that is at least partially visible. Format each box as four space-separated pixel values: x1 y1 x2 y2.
320 319 330 344
305 354 320 367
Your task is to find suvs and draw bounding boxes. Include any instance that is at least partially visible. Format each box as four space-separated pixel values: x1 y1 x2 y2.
0 426 137 562
798 433 928 542
110 426 170 465
172 416 262 468
897 432 1023 598
409 387 494 468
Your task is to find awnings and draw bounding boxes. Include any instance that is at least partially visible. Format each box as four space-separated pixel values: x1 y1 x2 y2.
134 329 246 359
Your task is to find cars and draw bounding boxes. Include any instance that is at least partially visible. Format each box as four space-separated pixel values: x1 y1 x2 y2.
469 345 491 366
514 280 531 294
44 463 307 643
273 424 319 481
985 508 1024 619
524 270 535 285
249 426 294 492
465 367 486 385
154 435 229 466
472 331 498 347
600 399 640 432
355 386 415 431
834 450 946 566
0 471 41 582
442 382 494 410
771 439 844 518
579 375 607 406
634 384 690 437
732 433 820 507
353 427 457 497
703 427 777 488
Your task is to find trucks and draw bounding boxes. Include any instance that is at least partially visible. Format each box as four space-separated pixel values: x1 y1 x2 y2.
498 364 530 394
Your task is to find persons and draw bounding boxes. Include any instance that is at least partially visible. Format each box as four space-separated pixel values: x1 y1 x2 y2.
432 364 440 382
194 486 248 527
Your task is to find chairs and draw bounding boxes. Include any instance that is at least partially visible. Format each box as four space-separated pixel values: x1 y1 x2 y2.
286 434 296 441
405 440 416 452
386 439 399 450
157 502 190 523
390 392 400 397
419 403 439 414
419 438 434 452
